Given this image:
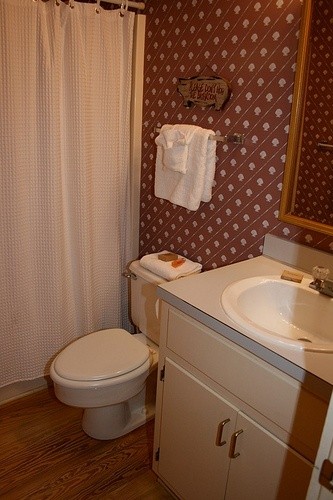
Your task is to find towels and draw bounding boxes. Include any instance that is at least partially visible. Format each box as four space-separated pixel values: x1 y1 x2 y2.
153 125 216 212
139 249 203 282
156 125 199 174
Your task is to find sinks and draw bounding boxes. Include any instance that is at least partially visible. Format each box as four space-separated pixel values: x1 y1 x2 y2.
220 275 333 353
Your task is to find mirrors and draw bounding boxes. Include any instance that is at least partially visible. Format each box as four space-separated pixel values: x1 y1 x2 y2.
277 1 333 237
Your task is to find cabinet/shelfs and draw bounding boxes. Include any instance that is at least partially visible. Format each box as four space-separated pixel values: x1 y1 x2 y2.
151 298 331 500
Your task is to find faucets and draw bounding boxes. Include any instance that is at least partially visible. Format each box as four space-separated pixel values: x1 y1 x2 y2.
308 265 331 292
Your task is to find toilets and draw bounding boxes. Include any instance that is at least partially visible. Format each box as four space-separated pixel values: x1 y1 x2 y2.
49 259 202 440
